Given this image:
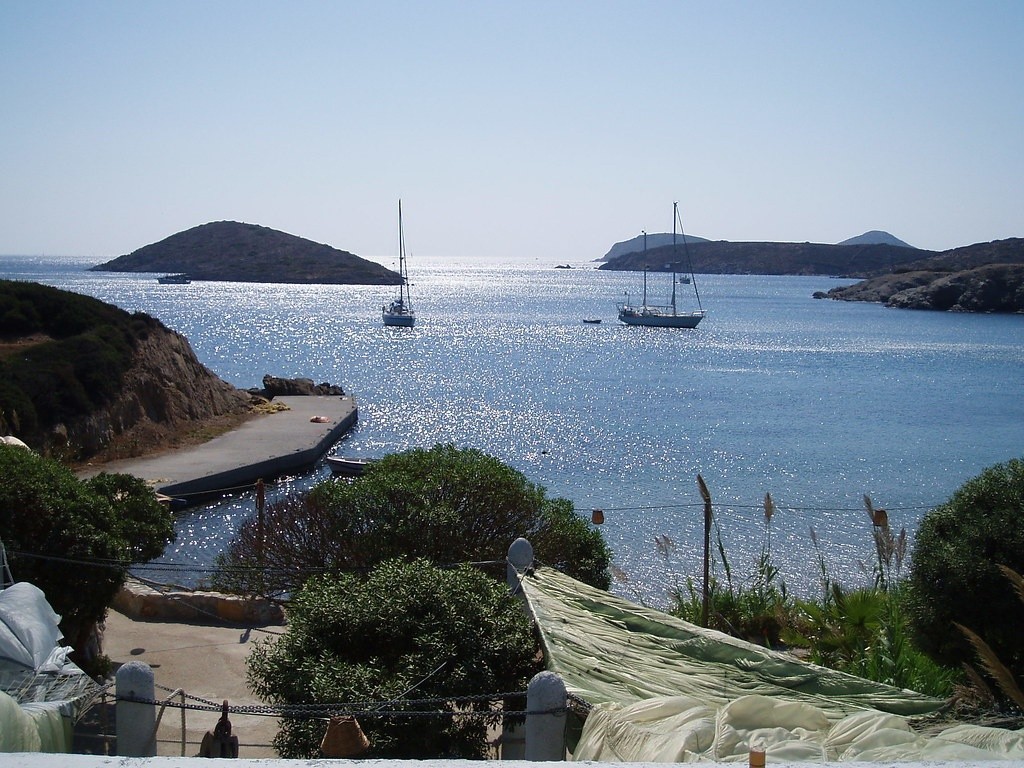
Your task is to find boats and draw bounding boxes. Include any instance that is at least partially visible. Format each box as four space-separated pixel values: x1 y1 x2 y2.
679 273 692 285
155 273 193 285
583 319 602 324
327 455 385 475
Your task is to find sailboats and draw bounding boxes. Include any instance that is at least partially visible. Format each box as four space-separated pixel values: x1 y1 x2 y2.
381 197 417 328
615 199 708 330
